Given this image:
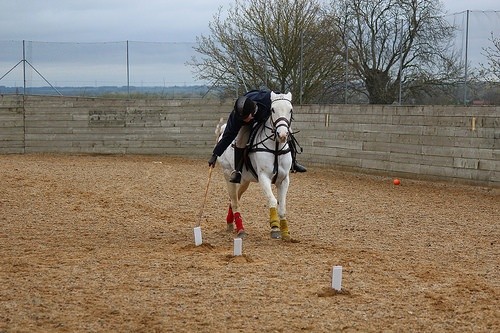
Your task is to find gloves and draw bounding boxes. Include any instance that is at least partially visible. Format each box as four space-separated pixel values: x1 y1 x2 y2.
208 154 217 168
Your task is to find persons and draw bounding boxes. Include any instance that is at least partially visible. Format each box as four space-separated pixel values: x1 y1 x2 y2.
208 89 307 183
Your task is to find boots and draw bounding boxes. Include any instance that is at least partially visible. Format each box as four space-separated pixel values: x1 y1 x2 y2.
287 139 307 172
229 142 245 183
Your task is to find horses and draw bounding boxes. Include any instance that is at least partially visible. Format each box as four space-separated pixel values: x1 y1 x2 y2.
215 91 292 241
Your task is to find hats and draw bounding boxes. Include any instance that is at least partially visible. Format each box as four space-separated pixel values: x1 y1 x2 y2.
235 96 254 120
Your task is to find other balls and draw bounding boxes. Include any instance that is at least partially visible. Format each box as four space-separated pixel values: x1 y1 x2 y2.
394 180 400 185
